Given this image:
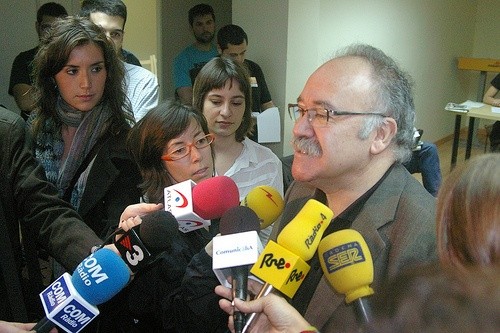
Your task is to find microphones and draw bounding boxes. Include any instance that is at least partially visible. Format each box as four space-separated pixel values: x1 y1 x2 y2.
29 175 374 333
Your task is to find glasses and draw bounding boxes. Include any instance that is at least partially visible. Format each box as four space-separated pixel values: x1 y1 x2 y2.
288 103 386 128
160 133 215 161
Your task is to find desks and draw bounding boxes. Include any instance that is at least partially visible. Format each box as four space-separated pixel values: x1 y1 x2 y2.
444 100 500 171
458 58 500 144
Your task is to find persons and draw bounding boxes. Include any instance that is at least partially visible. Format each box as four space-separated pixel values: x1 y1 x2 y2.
120 96 241 333
80 0 160 128
267 45 440 332
8 2 68 122
0 320 58 333
216 24 275 148
0 104 164 333
171 3 218 106
193 57 284 248
404 111 442 198
216 274 500 333
483 73 500 154
26 15 164 331
442 154 500 333
